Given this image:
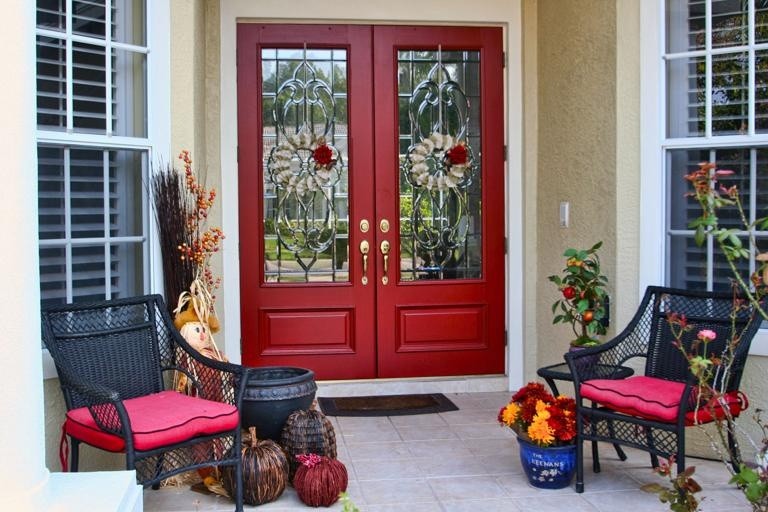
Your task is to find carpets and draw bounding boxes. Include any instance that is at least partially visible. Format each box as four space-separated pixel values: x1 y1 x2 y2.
317 393 459 417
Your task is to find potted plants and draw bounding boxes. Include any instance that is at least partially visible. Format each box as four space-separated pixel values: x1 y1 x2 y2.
546 240 610 367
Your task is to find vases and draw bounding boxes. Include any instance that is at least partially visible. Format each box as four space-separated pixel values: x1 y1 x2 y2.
516 435 577 488
234 365 318 441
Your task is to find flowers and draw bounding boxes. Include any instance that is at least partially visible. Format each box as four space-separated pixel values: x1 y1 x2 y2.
497 382 591 447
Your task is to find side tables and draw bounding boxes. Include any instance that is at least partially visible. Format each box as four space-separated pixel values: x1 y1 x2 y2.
537 362 634 472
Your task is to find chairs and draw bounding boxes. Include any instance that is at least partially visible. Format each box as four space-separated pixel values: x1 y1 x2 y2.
564 285 768 502
41 293 253 511
